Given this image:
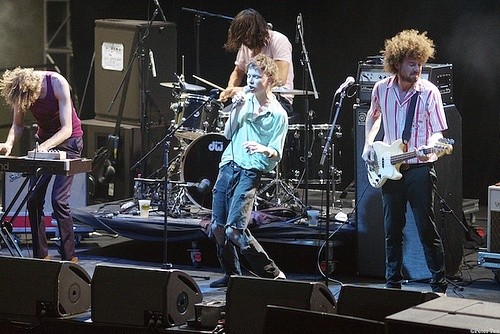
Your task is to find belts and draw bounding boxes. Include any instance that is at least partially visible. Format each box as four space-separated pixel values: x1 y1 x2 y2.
400 164 426 170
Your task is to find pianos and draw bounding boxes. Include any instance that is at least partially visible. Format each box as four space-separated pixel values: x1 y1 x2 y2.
0 156 92 257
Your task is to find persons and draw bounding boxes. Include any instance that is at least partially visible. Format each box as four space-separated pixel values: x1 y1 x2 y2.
218 9 294 115
362 30 449 298
209 53 288 289
0 65 83 264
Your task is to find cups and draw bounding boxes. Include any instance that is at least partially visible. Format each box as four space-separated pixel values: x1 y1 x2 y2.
138 200 151 219
307 210 320 226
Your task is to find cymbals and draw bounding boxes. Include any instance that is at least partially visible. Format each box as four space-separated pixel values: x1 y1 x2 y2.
160 82 207 91
271 89 319 96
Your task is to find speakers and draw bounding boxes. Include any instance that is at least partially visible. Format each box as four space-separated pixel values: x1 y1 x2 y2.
226 274 440 334
0 255 204 328
352 104 465 281
487 184 500 254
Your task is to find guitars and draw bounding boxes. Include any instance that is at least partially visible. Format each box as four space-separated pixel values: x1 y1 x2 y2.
365 137 453 189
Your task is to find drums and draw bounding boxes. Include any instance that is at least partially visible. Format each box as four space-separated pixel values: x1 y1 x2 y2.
176 94 224 140
252 170 277 210
280 124 342 185
180 132 232 212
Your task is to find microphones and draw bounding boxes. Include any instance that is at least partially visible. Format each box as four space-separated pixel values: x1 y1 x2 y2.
153 0 167 23
197 179 210 192
336 76 355 94
232 85 251 110
295 14 300 43
267 23 272 29
173 73 186 91
149 50 156 77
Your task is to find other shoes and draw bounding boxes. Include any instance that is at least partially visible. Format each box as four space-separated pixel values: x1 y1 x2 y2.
210 274 228 288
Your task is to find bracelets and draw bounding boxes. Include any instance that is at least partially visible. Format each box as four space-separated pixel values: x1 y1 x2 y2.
267 148 275 158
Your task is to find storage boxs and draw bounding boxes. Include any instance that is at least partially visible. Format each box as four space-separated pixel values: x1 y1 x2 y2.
477 251 500 268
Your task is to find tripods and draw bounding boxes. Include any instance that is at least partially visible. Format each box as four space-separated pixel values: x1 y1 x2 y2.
113 83 220 217
254 23 355 226
